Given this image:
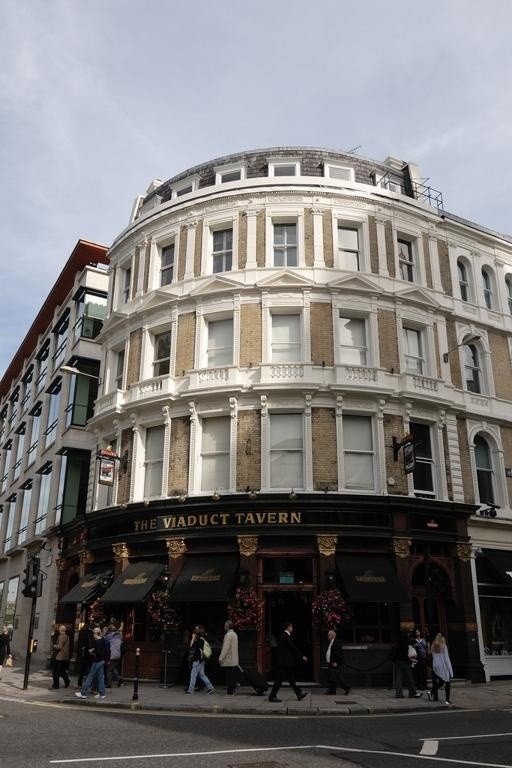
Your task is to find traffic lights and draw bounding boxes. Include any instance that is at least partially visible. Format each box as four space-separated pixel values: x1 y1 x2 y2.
21 556 40 598
21 558 44 599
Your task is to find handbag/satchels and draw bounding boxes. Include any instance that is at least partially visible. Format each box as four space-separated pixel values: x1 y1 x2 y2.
4 654 13 667
52 635 69 661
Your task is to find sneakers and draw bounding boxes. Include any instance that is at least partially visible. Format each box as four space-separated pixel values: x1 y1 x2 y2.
95 694 106 700
75 692 88 699
206 688 215 695
184 692 193 696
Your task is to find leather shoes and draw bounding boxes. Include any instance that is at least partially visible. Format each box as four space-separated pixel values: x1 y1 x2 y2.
298 692 308 701
65 679 71 688
345 686 353 695
48 686 57 690
269 697 282 702
427 691 433 702
445 701 455 705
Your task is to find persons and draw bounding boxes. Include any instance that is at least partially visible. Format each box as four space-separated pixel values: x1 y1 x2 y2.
395 627 423 698
218 620 241 696
78 623 91 690
412 628 427 690
427 632 453 705
75 627 106 699
103 623 124 689
185 623 204 692
269 620 308 702
492 613 504 652
48 625 71 690
324 630 352 695
184 623 216 696
0 631 13 665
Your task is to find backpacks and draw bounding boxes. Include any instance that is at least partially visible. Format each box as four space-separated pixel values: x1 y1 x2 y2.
199 637 212 658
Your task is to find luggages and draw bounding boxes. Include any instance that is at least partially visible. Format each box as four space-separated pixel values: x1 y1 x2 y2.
237 665 267 696
